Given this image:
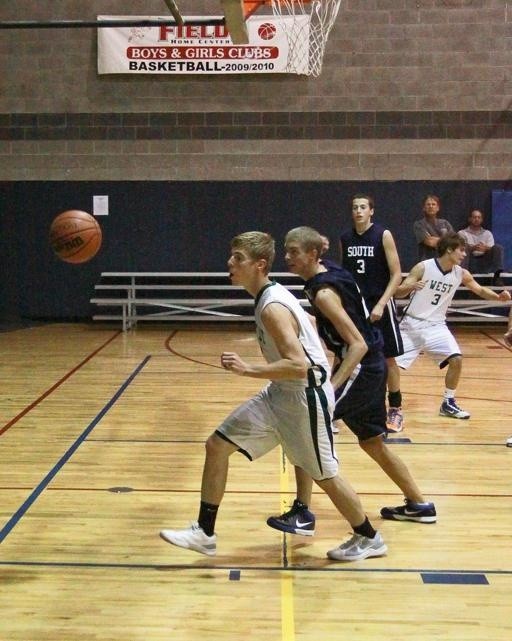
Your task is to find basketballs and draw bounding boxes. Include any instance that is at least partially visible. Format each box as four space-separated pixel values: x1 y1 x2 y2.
48 210 103 265
257 22 277 40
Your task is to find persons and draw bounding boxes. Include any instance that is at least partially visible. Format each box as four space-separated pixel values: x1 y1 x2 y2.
159 229 386 562
267 224 438 538
455 210 504 287
393 234 512 420
319 192 406 433
503 301 512 447
411 196 459 262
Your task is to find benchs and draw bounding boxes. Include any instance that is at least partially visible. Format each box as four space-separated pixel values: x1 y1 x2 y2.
93 267 511 333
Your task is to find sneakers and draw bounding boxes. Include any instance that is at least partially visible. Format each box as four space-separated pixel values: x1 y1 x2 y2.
267 500 315 538
439 398 470 420
332 425 340 434
327 530 388 561
160 520 218 558
385 405 404 433
380 499 436 524
505 435 512 448
